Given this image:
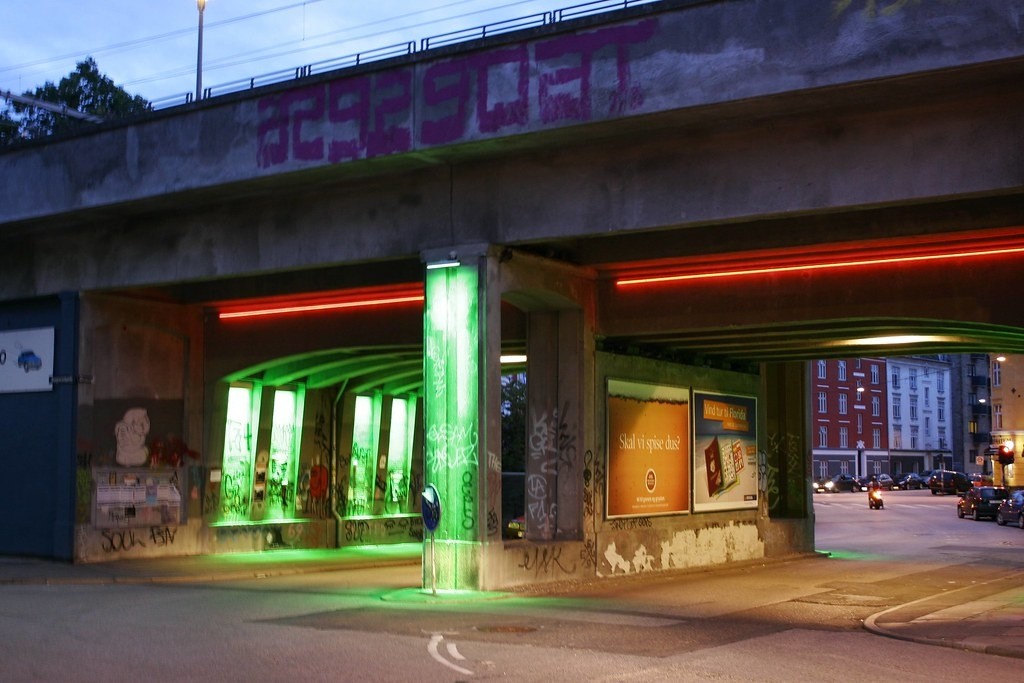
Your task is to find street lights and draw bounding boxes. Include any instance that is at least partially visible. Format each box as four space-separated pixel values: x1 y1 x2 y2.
194 1 206 100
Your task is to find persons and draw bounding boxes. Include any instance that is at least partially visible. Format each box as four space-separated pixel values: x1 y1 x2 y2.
869 475 881 493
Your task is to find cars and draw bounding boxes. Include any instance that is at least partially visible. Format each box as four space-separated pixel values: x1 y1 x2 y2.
919 470 933 489
995 490 1024 529
857 473 893 492
893 473 925 490
957 487 1010 521
968 475 993 489
815 474 860 494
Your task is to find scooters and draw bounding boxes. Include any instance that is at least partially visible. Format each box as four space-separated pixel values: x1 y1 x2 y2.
869 486 883 509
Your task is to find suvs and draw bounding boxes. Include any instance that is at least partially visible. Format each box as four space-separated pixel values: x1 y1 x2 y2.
928 469 974 496
